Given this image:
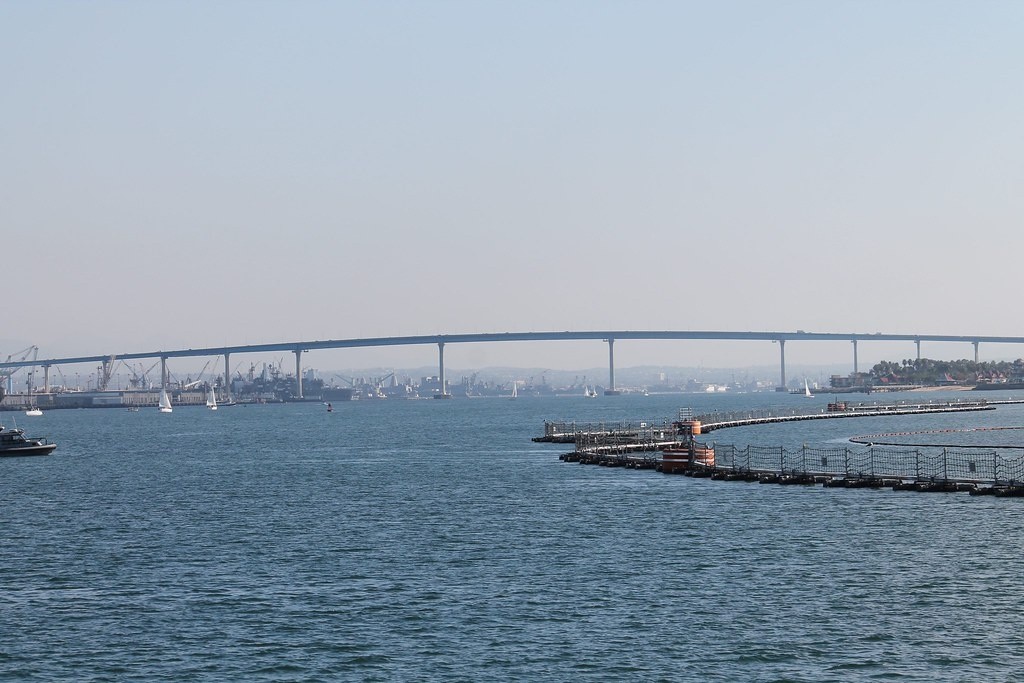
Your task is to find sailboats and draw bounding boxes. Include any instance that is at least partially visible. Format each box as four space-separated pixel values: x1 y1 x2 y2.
584 386 598 397
508 381 519 401
206 383 218 411
158 388 173 413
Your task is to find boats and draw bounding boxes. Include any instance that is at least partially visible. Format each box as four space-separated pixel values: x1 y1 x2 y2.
124 404 140 412
327 402 333 412
0 424 59 456
